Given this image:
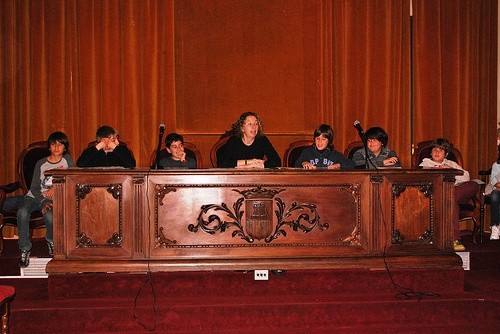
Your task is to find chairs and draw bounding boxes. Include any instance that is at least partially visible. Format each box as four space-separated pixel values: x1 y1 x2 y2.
478 168 492 241
210 135 232 168
411 140 480 245
150 142 203 168
343 141 366 160
283 140 315 168
0 141 70 252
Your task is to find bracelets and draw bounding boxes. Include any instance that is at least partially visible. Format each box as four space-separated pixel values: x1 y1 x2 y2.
245 159 247 164
182 160 187 162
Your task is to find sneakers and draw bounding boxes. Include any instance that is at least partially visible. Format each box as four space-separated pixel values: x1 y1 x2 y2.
454 239 465 251
490 225 500 240
19 250 30 268
48 242 54 256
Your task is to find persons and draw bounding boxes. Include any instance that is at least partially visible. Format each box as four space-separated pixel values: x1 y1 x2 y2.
352 127 401 170
16 131 75 267
153 133 198 169
295 124 355 170
419 138 470 250
76 125 136 168
218 112 287 169
485 143 500 240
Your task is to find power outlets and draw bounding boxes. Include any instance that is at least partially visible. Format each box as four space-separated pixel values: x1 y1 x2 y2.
254 270 269 280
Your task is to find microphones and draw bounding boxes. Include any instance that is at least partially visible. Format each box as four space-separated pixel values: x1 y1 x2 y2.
159 123 165 136
354 120 364 135
